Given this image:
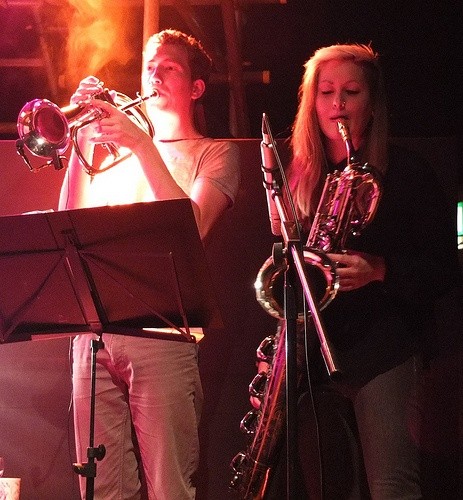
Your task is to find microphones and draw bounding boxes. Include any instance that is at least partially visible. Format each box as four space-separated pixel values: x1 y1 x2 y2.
260 116 281 235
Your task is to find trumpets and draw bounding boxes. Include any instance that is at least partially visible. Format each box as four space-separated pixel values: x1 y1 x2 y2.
14 88 162 177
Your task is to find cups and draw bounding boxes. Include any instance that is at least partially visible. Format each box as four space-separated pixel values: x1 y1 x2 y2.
0 478 21 500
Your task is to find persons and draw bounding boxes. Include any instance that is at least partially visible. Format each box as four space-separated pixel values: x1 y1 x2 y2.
57 30 242 500
283 43 463 500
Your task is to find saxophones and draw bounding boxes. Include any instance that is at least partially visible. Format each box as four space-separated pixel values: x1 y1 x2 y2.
226 118 382 500
